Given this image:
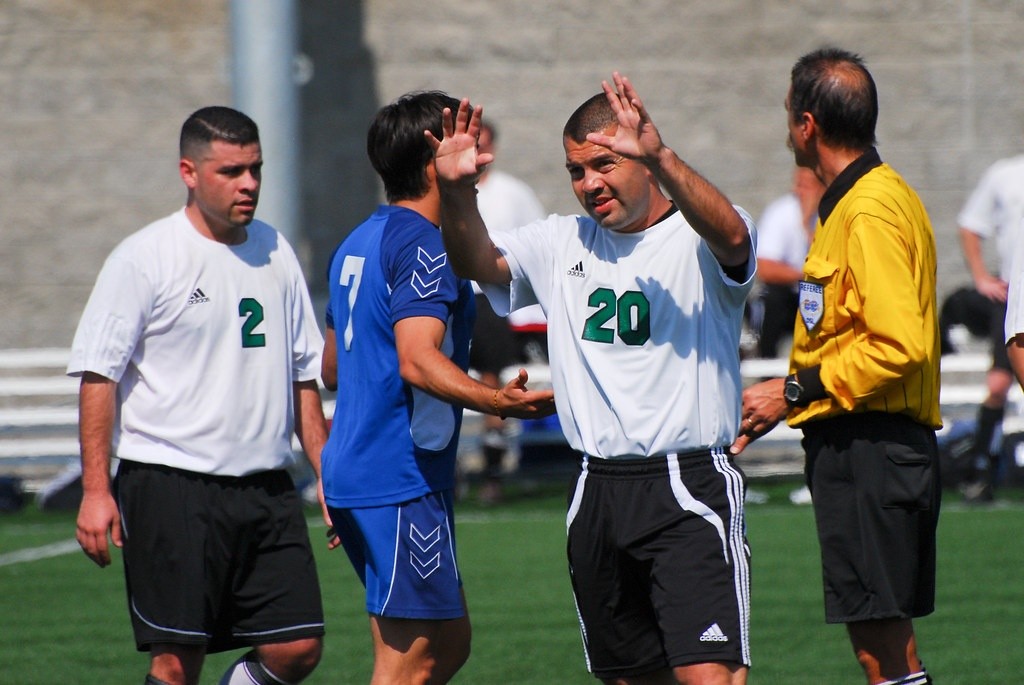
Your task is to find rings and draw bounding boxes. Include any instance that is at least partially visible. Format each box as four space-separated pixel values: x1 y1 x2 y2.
747 417 756 426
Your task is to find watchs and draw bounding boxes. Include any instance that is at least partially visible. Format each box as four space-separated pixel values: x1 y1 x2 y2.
785 374 805 410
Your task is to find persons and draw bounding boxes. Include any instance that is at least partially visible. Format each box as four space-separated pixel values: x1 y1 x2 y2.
423 70 756 685
66 105 341 685
460 123 546 463
321 90 557 685
956 151 1024 501
756 161 826 382
731 50 941 684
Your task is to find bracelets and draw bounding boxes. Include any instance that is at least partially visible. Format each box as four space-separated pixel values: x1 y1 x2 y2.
494 389 506 421
